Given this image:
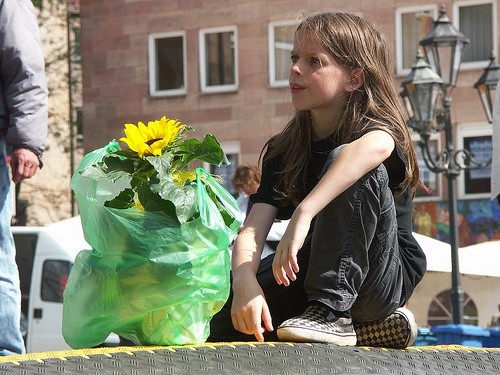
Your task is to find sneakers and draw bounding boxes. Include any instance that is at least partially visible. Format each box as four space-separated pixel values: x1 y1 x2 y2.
354 307 417 348
277 300 357 345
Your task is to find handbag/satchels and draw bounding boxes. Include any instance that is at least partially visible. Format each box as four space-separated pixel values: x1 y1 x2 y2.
61 140 242 349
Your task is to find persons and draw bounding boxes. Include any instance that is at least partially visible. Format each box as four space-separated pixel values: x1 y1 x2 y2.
229 163 262 201
208 11 426 350
0 0 49 357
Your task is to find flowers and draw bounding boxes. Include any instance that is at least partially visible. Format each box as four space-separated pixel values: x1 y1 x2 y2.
62 115 242 350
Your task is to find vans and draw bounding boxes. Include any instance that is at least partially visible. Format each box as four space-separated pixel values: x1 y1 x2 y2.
9 226 122 354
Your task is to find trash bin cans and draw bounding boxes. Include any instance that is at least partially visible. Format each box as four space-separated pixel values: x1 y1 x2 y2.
411 324 500 348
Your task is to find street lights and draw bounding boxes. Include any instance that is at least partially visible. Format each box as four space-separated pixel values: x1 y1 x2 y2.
398 4 500 325
